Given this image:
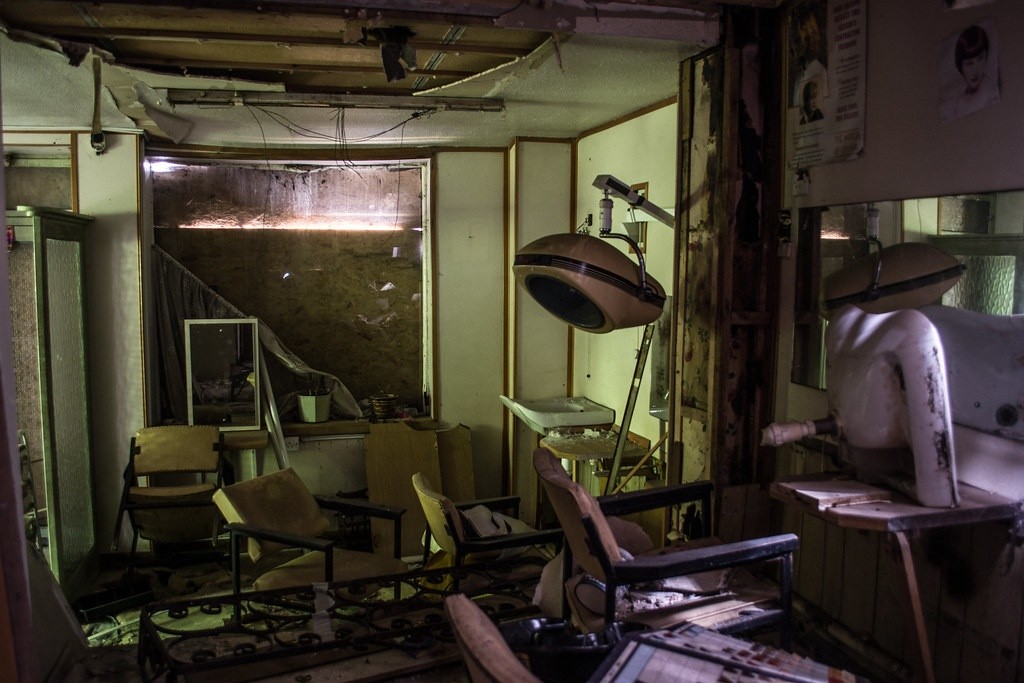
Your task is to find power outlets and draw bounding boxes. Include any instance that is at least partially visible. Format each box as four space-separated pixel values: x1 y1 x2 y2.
284 437 299 451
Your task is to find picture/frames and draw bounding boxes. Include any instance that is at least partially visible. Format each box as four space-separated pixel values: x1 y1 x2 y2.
147 148 443 425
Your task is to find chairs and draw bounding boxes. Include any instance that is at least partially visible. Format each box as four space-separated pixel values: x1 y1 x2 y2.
112 425 234 573
533 447 801 654
445 592 543 683
211 468 407 618
412 472 565 596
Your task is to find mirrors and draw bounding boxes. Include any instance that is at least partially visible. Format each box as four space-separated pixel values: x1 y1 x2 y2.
790 188 1024 445
184 319 261 431
